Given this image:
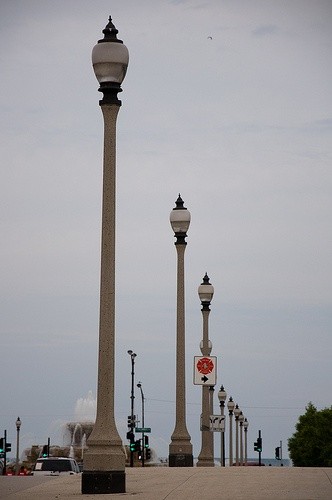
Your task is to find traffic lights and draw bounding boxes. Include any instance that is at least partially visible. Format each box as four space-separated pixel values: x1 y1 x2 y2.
43 445 47 458
254 437 262 452
136 440 140 452
127 432 132 439
130 442 137 452
0 438 3 453
128 415 135 427
5 443 11 452
145 436 148 448
146 448 150 460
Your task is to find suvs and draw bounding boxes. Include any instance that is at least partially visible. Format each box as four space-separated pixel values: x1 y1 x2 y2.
33 457 80 477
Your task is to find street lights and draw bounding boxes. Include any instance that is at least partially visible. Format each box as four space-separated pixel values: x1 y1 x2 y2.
196 272 215 467
168 192 193 467
227 396 235 466
137 384 144 467
200 340 214 415
15 416 22 475
234 404 240 466
218 384 227 467
238 411 244 466
127 349 137 467
243 418 248 466
81 14 129 494
